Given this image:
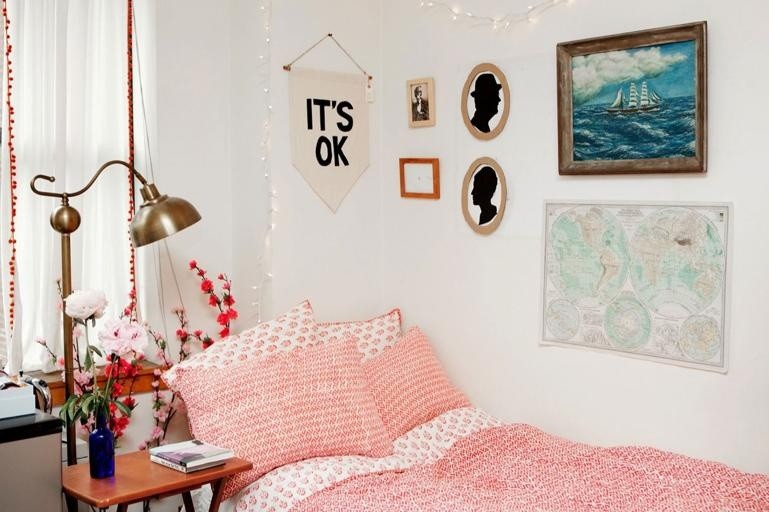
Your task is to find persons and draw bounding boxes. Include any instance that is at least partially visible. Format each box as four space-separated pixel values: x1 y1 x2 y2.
411 86 429 121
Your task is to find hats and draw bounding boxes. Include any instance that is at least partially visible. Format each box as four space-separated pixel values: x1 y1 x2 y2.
471 73 502 98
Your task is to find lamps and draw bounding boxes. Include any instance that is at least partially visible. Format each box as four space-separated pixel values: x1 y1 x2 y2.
29 160 201 512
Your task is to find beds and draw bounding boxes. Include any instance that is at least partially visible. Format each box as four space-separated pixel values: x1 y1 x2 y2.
200 406 769 511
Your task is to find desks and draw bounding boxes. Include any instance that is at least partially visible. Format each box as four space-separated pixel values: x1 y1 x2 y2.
61 439 253 511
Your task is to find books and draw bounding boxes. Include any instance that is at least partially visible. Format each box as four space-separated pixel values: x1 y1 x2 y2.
148 439 235 474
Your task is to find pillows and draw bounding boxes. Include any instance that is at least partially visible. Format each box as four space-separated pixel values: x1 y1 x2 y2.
315 306 402 361
179 340 394 503
160 298 319 396
363 325 472 443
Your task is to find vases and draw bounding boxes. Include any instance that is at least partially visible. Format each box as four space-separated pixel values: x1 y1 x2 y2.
87 402 117 480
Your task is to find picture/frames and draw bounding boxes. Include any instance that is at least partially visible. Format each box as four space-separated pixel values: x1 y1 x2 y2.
399 156 440 202
462 156 509 238
552 18 710 178
460 62 511 144
404 75 437 129
541 197 730 380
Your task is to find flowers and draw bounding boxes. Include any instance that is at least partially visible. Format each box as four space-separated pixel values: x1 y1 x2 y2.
37 256 239 448
65 287 145 416
